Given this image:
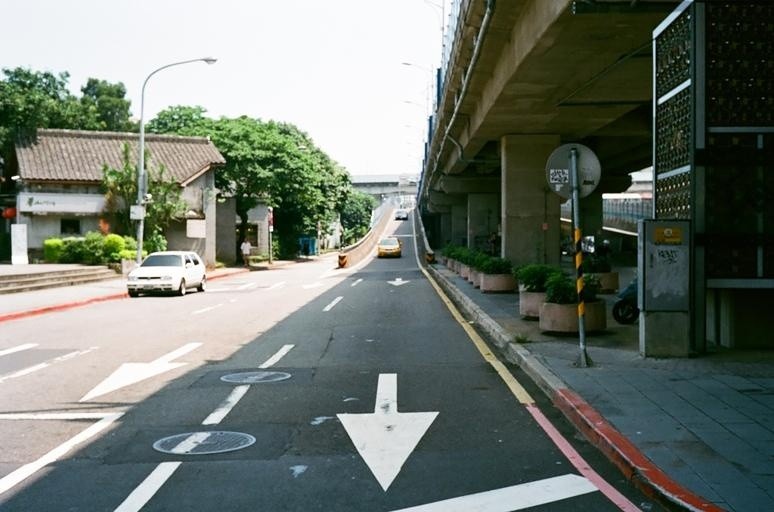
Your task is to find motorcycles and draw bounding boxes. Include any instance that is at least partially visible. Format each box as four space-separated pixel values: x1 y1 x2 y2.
609 269 640 327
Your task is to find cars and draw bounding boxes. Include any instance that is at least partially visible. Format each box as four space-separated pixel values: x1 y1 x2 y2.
377 235 402 258
394 208 409 221
126 249 207 301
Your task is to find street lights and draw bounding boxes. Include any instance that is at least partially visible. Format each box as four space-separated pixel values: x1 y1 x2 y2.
292 145 308 154
399 59 436 146
135 55 220 266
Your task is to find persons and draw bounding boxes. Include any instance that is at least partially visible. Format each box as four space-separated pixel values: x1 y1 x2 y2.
240 236 251 267
